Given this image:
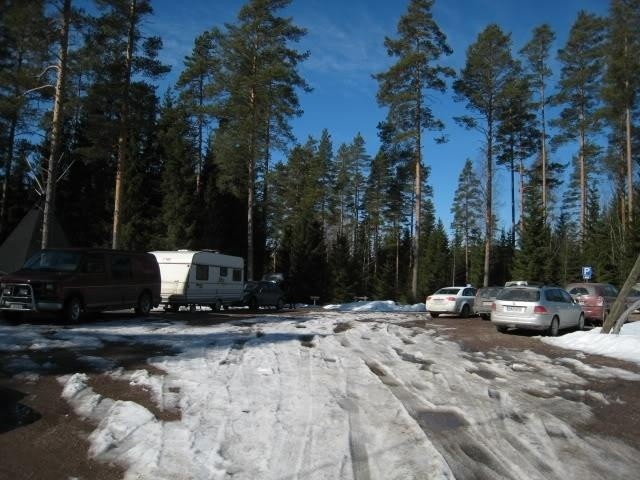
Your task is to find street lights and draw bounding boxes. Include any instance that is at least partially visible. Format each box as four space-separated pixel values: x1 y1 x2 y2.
452 243 458 286
270 244 276 273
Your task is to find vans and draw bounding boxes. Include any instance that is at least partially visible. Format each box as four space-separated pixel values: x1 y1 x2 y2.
0 248 161 324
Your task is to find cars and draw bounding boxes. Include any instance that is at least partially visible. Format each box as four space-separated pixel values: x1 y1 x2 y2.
223 273 288 310
425 280 619 336
